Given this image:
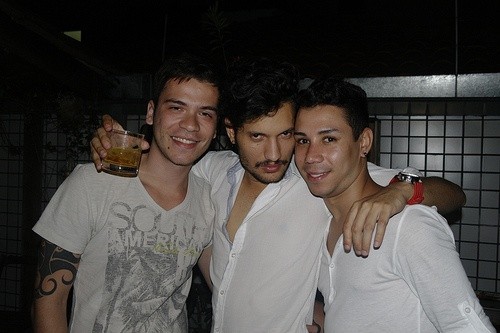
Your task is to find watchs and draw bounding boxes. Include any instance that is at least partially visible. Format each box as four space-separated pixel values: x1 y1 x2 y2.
387 165 424 205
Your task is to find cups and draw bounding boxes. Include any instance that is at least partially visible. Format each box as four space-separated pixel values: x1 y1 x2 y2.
101 128 145 177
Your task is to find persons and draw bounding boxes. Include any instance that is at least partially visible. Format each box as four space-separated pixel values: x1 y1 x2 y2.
90 67 466 333
292 77 499 332
29 59 215 333
